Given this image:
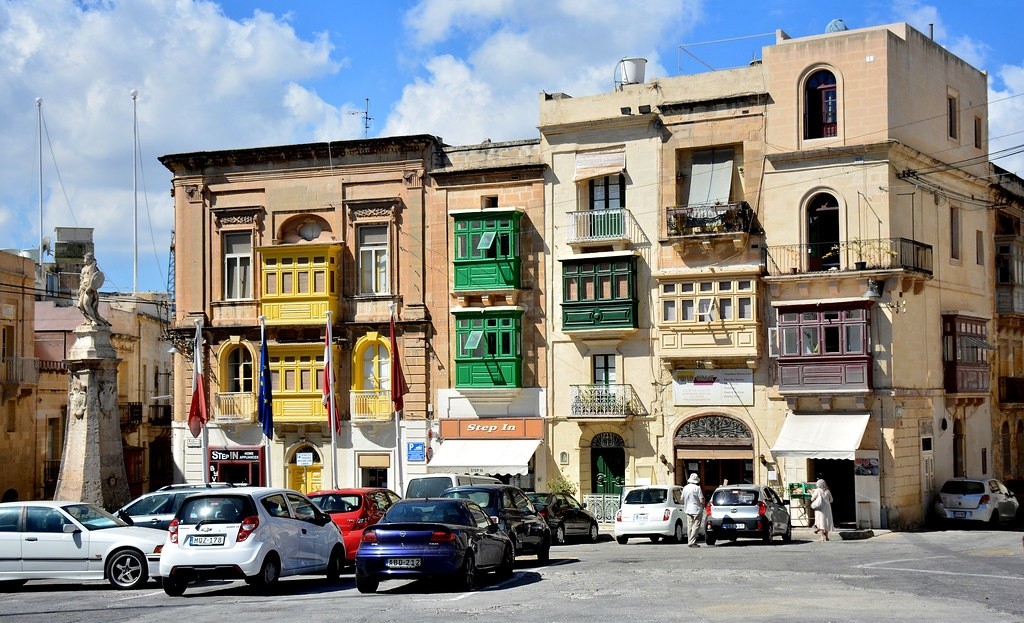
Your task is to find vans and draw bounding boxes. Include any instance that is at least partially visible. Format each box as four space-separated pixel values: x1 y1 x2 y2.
403 475 511 511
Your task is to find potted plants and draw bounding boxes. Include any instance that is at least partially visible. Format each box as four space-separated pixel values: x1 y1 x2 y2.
821 236 898 270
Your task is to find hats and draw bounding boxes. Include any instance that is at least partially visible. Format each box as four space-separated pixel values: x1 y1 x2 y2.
688 473 700 483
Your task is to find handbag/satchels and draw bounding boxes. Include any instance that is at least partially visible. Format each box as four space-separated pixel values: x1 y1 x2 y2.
811 489 826 510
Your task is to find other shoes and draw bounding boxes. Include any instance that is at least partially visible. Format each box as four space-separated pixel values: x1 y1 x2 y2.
689 544 700 548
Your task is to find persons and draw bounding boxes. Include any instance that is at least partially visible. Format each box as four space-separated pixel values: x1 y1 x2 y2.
810 479 833 541
681 473 705 548
77 252 113 326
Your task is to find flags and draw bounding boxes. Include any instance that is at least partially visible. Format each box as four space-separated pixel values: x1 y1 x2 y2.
188 330 208 438
322 317 341 436
257 324 273 441
390 314 409 412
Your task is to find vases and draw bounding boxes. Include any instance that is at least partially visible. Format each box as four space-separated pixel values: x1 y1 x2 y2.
790 268 797 275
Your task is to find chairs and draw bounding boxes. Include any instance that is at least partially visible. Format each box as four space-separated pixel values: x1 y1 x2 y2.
729 493 740 503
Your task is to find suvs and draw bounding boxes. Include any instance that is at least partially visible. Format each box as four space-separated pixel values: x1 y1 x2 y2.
77 480 256 530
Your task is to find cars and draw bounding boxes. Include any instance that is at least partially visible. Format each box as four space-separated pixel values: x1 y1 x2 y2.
935 477 1020 530
511 492 599 545
355 497 516 594
0 502 173 592
293 488 412 574
614 485 707 544
159 488 348 597
431 484 552 564
703 484 793 545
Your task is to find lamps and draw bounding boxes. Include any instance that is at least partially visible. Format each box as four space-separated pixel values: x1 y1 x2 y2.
862 279 881 297
620 107 635 116
738 166 744 177
638 105 654 114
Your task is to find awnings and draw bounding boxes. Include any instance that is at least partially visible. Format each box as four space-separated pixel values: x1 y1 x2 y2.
770 414 870 460
427 440 540 476
